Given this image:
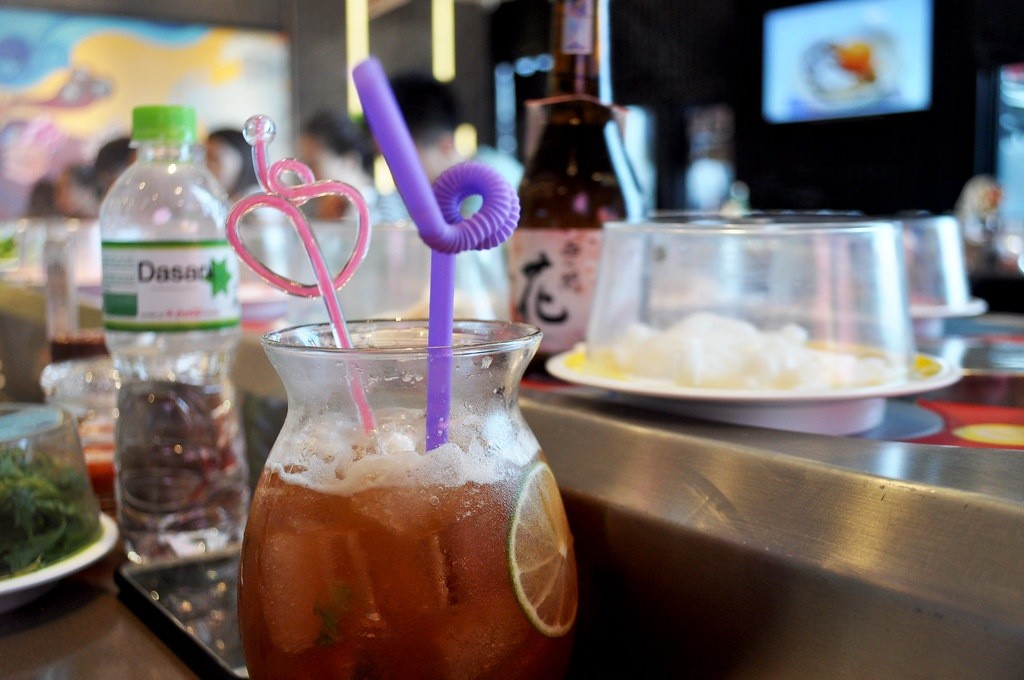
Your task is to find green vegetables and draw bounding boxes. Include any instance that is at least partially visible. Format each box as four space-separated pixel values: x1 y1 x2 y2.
0 438 102 581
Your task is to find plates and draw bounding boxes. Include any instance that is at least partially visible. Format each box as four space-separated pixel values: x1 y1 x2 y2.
544 349 964 437
777 302 988 341
0 510 119 614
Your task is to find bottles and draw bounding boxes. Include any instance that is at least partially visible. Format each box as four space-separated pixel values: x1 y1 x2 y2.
99 106 251 563
505 3 645 385
237 317 578 680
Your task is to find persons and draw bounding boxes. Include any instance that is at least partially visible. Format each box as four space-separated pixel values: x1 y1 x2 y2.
205 130 284 287
363 73 525 316
301 115 388 308
55 139 180 234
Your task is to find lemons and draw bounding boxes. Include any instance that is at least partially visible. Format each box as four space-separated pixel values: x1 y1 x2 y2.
506 460 576 634
